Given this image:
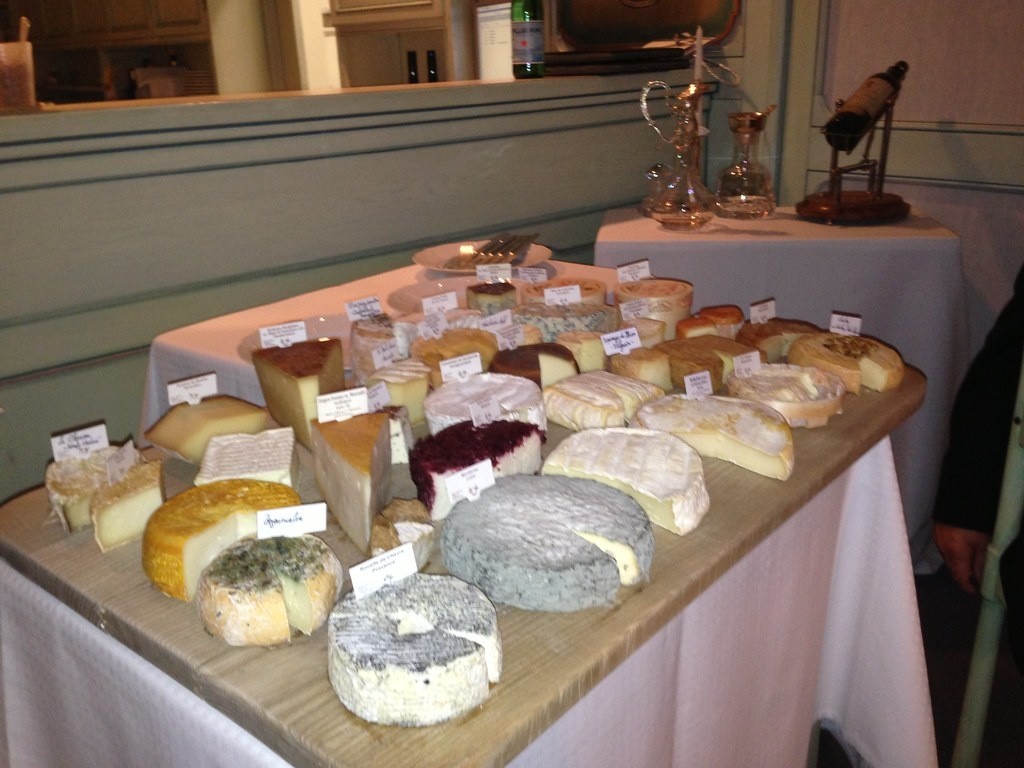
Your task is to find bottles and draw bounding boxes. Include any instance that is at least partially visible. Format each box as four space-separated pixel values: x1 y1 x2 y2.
511 0 546 80
713 110 776 220
824 60 909 152
427 49 438 82
407 51 419 84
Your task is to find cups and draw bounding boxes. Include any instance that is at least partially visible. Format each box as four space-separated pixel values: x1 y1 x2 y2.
0 41 37 116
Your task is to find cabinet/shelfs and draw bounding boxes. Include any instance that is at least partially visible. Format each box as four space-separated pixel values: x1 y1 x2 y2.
1 1 275 105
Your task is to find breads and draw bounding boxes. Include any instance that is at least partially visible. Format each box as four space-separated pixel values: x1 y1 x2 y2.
42 278 907 729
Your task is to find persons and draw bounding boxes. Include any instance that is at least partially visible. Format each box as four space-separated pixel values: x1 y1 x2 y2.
928 263 1024 686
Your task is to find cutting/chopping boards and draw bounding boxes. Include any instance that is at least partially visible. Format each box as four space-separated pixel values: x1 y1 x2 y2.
0 363 928 768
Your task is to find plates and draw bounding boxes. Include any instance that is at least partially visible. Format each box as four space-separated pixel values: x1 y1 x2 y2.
412 239 553 276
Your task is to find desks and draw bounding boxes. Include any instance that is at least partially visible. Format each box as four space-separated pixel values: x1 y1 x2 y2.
596 201 962 554
0 360 949 767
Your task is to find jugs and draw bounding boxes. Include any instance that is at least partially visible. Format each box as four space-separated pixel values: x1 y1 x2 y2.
636 79 716 232
